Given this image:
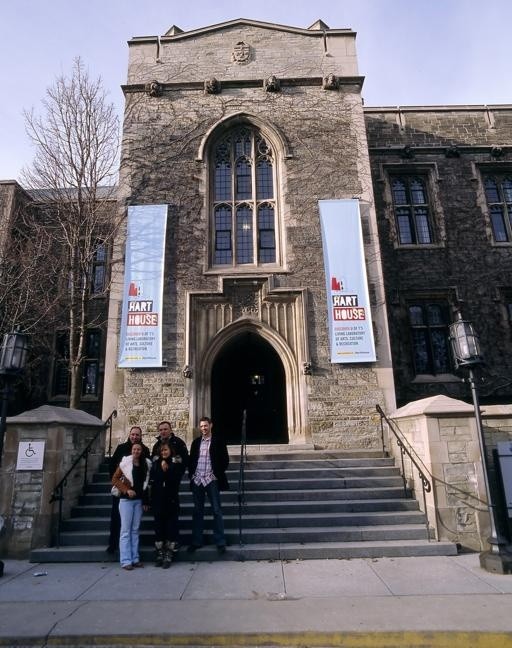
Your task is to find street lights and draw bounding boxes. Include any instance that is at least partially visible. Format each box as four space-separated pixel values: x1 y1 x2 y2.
447 310 512 575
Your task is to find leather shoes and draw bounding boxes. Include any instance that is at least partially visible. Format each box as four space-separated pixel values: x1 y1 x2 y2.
218 545 225 555
125 562 143 570
187 544 203 553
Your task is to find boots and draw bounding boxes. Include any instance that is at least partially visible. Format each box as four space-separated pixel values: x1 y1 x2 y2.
155 547 174 568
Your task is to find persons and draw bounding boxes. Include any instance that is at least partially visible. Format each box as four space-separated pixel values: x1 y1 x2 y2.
150 421 188 484
148 440 186 569
105 426 150 554
187 417 229 556
111 441 152 570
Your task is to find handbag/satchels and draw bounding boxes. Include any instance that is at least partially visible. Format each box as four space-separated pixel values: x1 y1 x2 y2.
110 477 125 498
